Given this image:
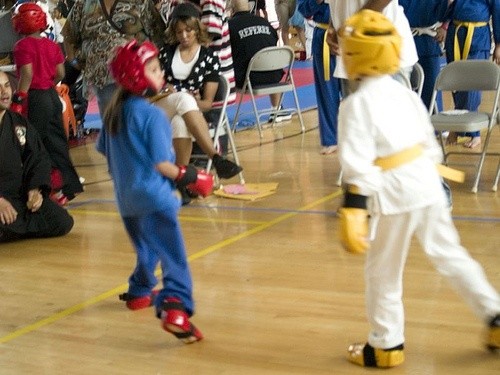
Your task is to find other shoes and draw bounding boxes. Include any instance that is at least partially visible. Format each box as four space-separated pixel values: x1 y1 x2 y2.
161 297 203 344
344 340 405 369
175 182 196 204
482 313 500 350
48 190 68 206
215 159 244 179
126 289 162 310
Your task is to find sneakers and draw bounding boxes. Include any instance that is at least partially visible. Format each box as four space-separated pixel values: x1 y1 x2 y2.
268 104 291 122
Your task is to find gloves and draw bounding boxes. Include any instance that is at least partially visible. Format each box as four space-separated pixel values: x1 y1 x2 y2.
334 185 371 256
175 164 214 199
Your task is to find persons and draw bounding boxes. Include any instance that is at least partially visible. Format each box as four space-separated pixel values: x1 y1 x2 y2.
0 0 500 156
335 10 500 369
94 40 215 346
10 4 84 206
154 3 242 205
0 70 74 241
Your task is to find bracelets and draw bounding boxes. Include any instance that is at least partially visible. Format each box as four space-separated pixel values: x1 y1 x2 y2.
440 23 448 31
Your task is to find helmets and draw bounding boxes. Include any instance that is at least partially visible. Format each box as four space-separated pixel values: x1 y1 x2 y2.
338 9 400 79
11 2 46 34
106 38 167 97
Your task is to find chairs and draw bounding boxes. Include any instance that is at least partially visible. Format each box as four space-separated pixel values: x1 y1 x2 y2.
190 75 245 199
334 59 500 193
231 46 304 144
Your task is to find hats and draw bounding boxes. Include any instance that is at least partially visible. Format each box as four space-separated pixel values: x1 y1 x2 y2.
174 3 199 19
